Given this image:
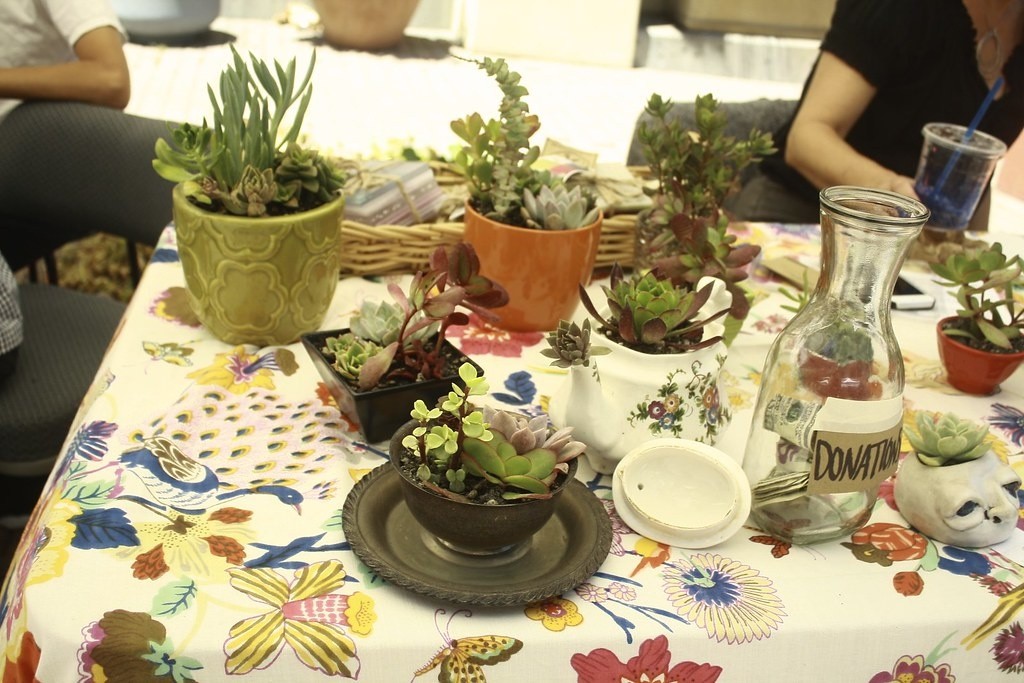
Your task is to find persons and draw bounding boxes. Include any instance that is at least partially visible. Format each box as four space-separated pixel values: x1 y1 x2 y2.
0 0 252 277
720 0 1024 236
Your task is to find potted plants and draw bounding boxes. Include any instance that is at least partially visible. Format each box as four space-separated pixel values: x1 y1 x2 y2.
895 411 1021 550
782 267 873 399
927 243 1024 397
447 51 605 334
389 361 586 559
297 241 510 447
538 260 736 476
634 92 780 351
152 39 352 347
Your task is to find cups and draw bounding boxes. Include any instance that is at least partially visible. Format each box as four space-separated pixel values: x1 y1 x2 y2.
909 122 1006 249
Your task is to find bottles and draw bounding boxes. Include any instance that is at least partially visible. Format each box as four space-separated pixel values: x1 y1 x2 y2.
737 183 934 547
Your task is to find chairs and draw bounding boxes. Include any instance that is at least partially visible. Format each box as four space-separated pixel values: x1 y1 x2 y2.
626 100 804 178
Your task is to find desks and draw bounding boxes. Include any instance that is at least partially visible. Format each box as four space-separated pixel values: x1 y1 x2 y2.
0 219 1024 681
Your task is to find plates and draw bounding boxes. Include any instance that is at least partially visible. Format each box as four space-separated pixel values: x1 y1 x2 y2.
341 456 615 607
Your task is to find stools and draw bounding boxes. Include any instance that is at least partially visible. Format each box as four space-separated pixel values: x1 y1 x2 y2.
1 220 139 288
2 286 128 544
